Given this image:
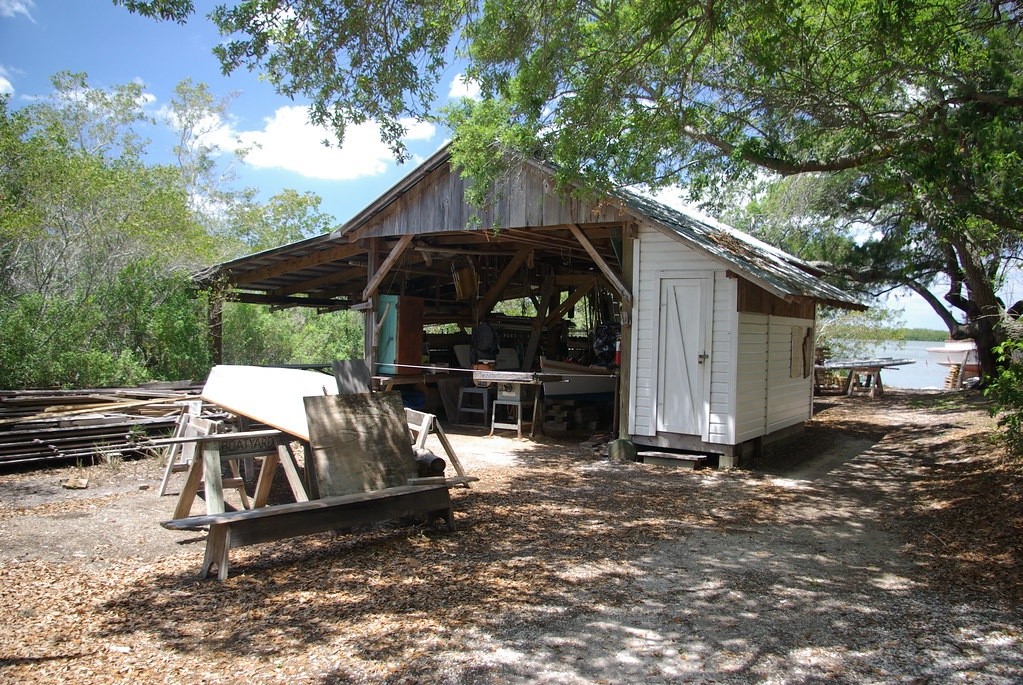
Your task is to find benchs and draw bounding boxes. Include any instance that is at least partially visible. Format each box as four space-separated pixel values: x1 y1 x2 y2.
160 476 480 583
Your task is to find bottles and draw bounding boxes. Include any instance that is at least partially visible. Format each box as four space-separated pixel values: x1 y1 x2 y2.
499 331 531 346
